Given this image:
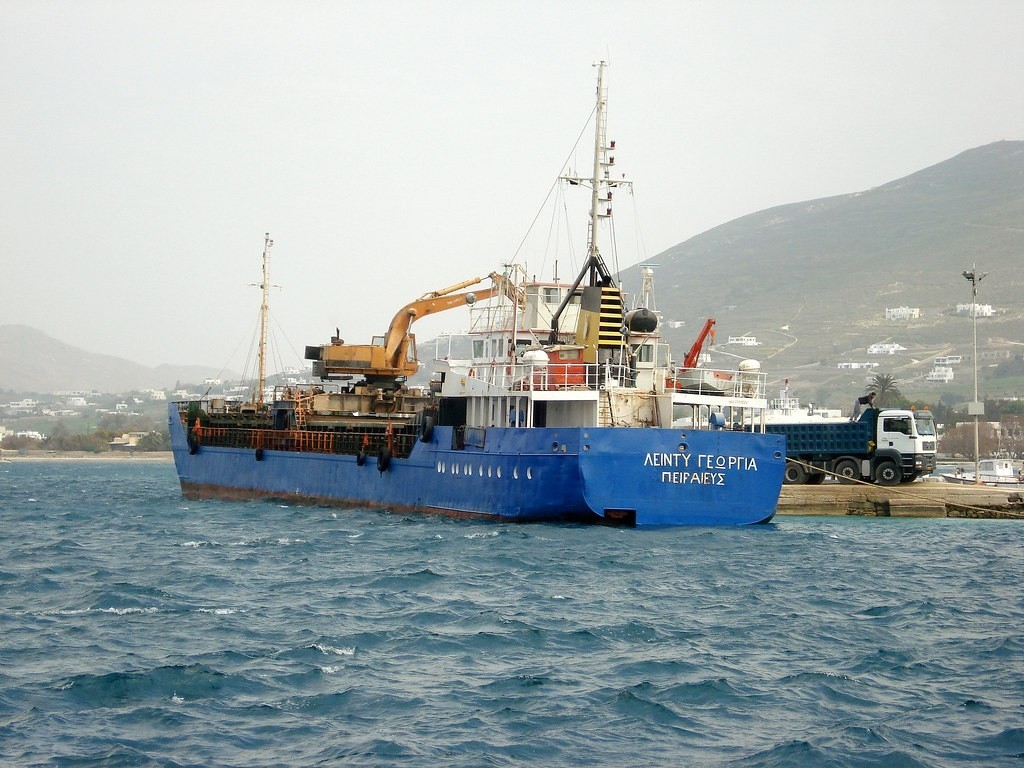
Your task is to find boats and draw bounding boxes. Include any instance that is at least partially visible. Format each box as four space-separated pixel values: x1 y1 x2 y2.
939 458 1024 490
165 60 786 526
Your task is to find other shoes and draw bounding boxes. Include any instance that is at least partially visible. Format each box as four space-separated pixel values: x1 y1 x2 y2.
849 419 857 423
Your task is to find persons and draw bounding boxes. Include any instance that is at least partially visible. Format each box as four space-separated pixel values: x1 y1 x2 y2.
848 391 876 423
508 405 521 428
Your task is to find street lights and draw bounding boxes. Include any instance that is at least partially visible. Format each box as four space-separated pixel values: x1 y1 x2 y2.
963 261 990 485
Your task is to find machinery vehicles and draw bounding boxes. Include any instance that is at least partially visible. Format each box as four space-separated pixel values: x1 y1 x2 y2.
665 316 718 388
294 271 526 416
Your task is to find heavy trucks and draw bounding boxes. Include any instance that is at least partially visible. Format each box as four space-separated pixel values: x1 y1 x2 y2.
733 407 937 486
